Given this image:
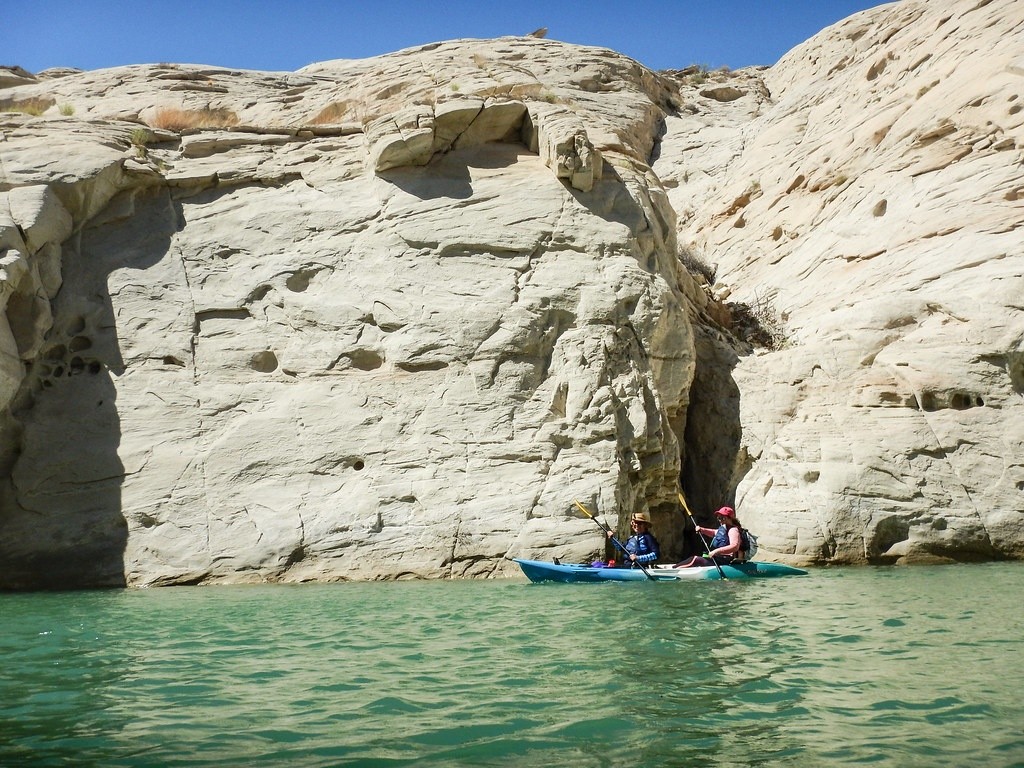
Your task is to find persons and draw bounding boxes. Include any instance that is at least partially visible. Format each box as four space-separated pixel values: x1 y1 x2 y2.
592 513 658 569
675 507 742 568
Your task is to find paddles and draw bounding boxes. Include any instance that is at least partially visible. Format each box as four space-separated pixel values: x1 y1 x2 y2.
677 492 726 580
573 498 656 581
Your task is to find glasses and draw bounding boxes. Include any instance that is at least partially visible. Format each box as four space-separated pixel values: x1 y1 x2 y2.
717 516 728 520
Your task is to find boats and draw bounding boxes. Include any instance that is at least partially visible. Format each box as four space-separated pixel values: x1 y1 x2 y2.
512 557 808 591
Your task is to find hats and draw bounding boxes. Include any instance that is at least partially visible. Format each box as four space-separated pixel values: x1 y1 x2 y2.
632 513 653 529
714 507 734 520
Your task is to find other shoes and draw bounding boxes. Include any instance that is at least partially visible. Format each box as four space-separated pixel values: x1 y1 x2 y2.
554 557 560 565
